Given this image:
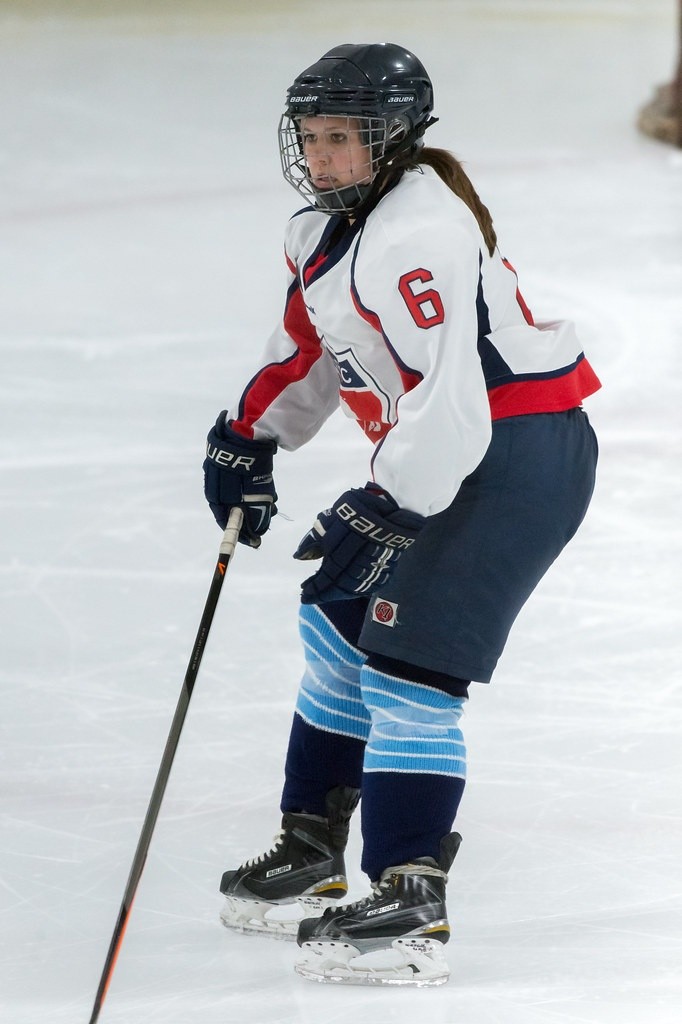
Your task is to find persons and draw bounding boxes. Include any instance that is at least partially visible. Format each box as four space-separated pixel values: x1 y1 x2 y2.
202 40 603 985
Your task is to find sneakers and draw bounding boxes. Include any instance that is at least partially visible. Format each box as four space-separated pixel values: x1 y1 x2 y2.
218 786 360 944
294 831 462 987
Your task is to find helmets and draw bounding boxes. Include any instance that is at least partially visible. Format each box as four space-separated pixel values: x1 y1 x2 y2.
279 43 434 216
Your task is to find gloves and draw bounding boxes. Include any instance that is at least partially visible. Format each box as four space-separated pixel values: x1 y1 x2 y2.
203 409 279 549
293 483 427 605
